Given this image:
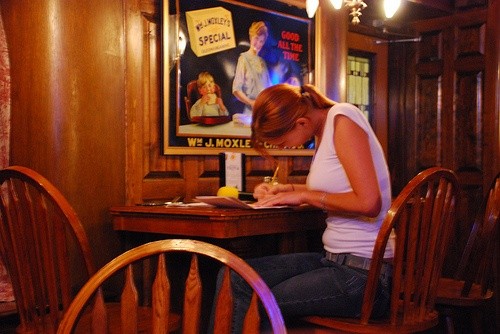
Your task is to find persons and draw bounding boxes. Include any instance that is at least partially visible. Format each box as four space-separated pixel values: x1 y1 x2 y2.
206 83 394 334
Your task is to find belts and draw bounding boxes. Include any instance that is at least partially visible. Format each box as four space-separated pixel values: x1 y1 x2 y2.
325 252 393 278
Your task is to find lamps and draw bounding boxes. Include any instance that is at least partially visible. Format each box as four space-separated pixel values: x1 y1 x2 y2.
306 0 401 24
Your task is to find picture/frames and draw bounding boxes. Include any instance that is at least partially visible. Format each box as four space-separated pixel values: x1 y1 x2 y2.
162 0 322 156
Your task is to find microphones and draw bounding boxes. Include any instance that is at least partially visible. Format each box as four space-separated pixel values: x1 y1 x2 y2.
217 185 257 203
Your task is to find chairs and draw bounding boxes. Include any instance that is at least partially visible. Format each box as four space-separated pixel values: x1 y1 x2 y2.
52 237 287 334
298 164 500 334
0 164 181 334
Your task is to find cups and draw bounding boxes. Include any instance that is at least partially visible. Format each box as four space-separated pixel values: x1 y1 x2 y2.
264 176 279 190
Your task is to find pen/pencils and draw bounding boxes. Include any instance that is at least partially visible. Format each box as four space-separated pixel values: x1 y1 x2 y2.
269 165 280 186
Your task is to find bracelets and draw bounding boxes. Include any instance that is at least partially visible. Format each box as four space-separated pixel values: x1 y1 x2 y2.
319 192 328 211
290 183 295 190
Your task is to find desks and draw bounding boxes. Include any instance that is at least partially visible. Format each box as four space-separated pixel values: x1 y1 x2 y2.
108 203 327 309
